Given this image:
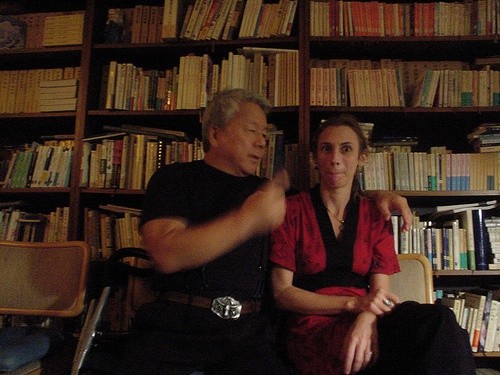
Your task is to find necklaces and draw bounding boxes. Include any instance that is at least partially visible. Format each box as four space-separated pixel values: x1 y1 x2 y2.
327 208 344 224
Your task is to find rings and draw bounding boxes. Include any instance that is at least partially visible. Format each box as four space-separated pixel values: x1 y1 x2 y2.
383 298 392 306
364 351 374 356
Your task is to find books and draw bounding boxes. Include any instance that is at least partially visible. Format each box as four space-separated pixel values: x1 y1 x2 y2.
0 0 500 375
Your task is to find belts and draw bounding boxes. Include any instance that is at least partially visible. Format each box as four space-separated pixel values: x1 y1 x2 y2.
159 291 263 320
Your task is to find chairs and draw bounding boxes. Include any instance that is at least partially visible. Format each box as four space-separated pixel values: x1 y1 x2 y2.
389 253 433 305
0 240 90 375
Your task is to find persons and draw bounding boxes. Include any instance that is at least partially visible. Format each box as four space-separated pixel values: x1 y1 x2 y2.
270 116 477 375
108 88 414 375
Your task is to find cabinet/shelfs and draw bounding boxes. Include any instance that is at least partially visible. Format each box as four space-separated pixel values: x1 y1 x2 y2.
0 0 500 375
